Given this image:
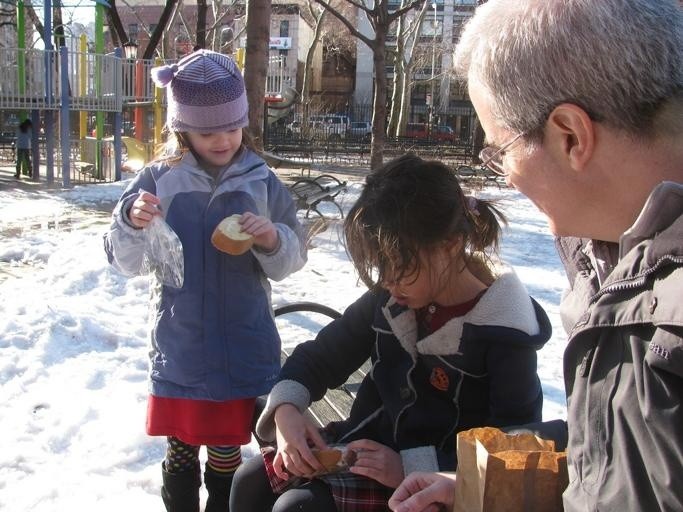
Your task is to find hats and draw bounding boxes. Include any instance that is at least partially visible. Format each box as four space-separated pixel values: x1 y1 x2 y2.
150 49 250 133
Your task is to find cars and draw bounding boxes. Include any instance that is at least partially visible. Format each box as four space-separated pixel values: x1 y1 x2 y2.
288 115 456 143
1 113 136 146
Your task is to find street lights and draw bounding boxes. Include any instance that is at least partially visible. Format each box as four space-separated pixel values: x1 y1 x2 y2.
428 0 436 141
123 35 140 139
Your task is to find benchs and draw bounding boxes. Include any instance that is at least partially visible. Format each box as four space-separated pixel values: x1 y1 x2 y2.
246 301 372 446
453 165 502 190
286 175 348 223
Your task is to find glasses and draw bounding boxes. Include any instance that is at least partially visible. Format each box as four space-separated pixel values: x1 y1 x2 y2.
480 129 531 178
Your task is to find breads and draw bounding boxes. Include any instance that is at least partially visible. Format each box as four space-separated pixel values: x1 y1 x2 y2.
301 446 356 479
210 213 253 257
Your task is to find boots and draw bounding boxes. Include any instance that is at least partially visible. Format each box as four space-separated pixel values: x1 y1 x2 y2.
161 459 202 512
204 461 242 512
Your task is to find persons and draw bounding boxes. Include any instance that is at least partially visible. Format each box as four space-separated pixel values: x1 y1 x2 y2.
104 48 309 512
228 154 553 511
13 119 33 180
387 0 683 512
